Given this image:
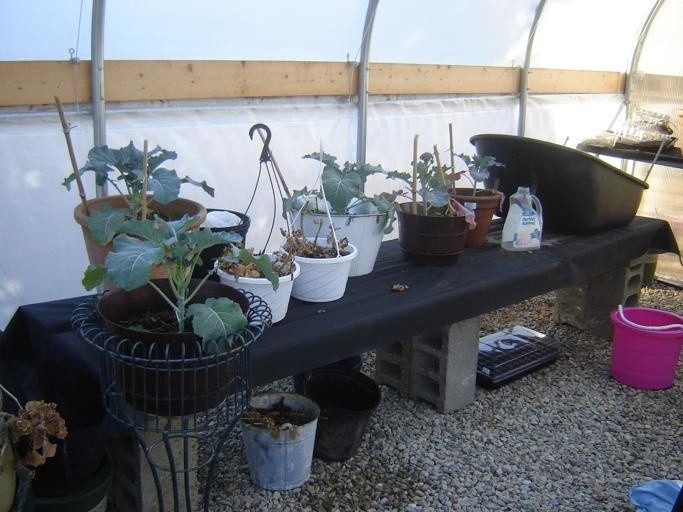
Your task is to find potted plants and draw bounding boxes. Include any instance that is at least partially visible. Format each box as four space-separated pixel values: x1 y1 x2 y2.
283 146 415 278
63 141 208 307
389 151 473 271
446 148 505 249
69 208 254 413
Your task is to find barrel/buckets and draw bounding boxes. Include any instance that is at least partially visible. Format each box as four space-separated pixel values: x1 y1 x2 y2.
287 195 388 277
301 365 382 463
190 208 251 281
239 393 321 491
608 303 683 390
396 201 466 266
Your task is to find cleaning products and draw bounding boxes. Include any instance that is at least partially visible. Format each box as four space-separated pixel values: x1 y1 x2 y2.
501 187 543 253
